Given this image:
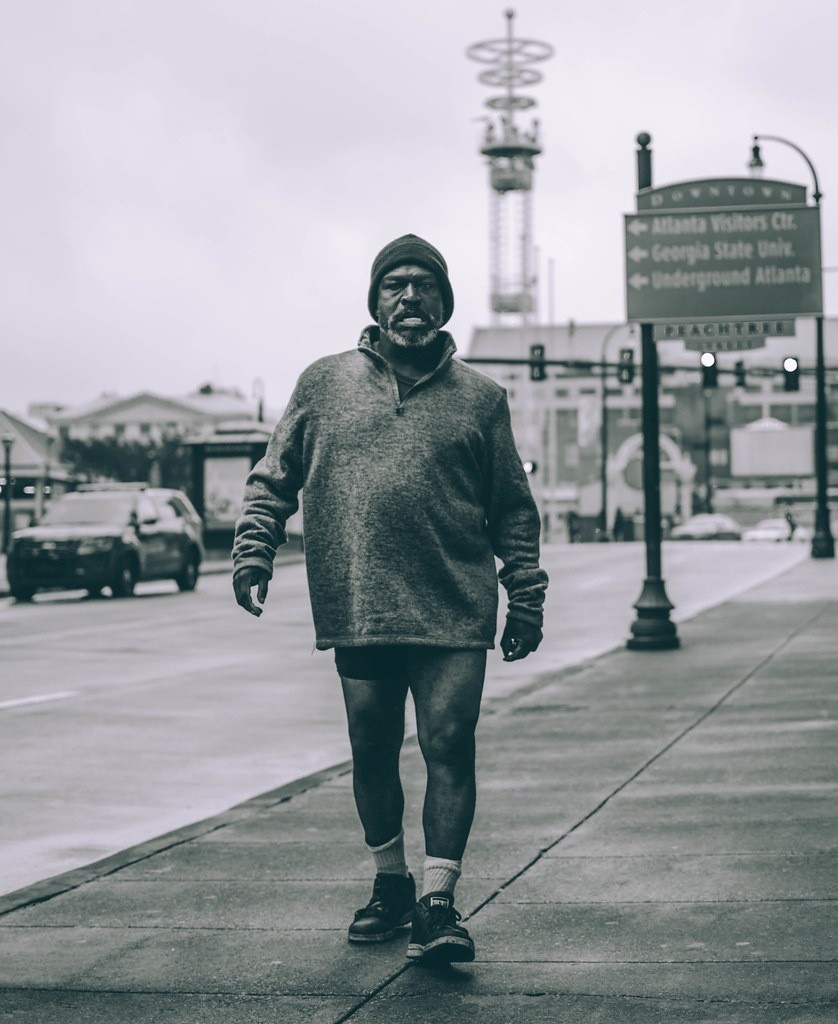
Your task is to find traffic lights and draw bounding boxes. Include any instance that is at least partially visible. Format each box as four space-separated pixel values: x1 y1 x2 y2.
702 353 716 388
783 356 799 392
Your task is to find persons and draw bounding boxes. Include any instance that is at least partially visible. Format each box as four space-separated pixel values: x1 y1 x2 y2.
230 234 549 969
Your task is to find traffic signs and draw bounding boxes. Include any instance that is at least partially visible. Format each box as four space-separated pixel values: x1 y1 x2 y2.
624 208 824 324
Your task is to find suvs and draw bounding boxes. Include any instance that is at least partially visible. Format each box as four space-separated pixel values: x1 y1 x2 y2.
6 483 206 601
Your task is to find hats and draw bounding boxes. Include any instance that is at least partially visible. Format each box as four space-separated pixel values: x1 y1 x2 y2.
368 234 454 329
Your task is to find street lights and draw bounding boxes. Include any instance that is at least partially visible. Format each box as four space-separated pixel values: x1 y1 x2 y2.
746 133 831 560
2 429 14 554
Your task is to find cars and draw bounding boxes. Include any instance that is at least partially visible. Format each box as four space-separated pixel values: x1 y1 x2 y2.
741 518 792 542
672 512 742 540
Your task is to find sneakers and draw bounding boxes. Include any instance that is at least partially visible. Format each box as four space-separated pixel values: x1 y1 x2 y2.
348 872 417 942
406 891 475 962
